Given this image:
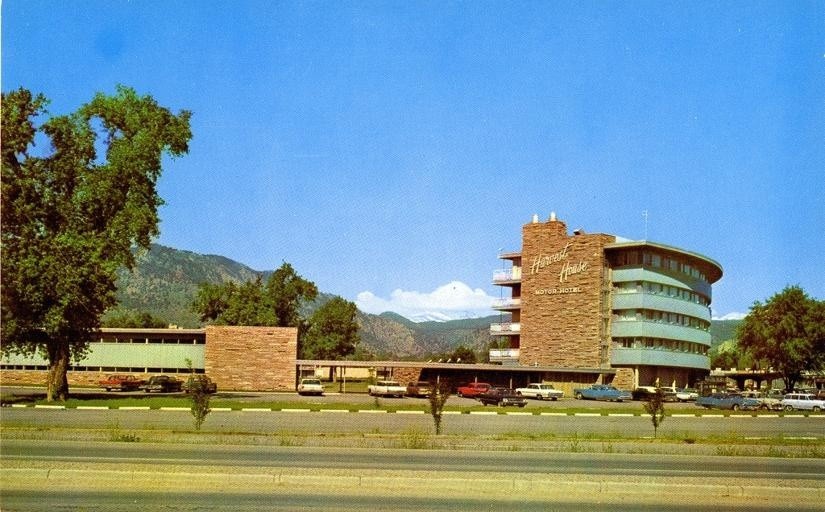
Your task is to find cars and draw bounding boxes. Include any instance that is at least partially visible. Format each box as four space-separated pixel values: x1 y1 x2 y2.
367 381 439 397
574 383 825 412
457 383 564 407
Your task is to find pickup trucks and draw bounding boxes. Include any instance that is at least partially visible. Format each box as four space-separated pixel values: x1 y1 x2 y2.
99 375 216 392
298 379 324 396
